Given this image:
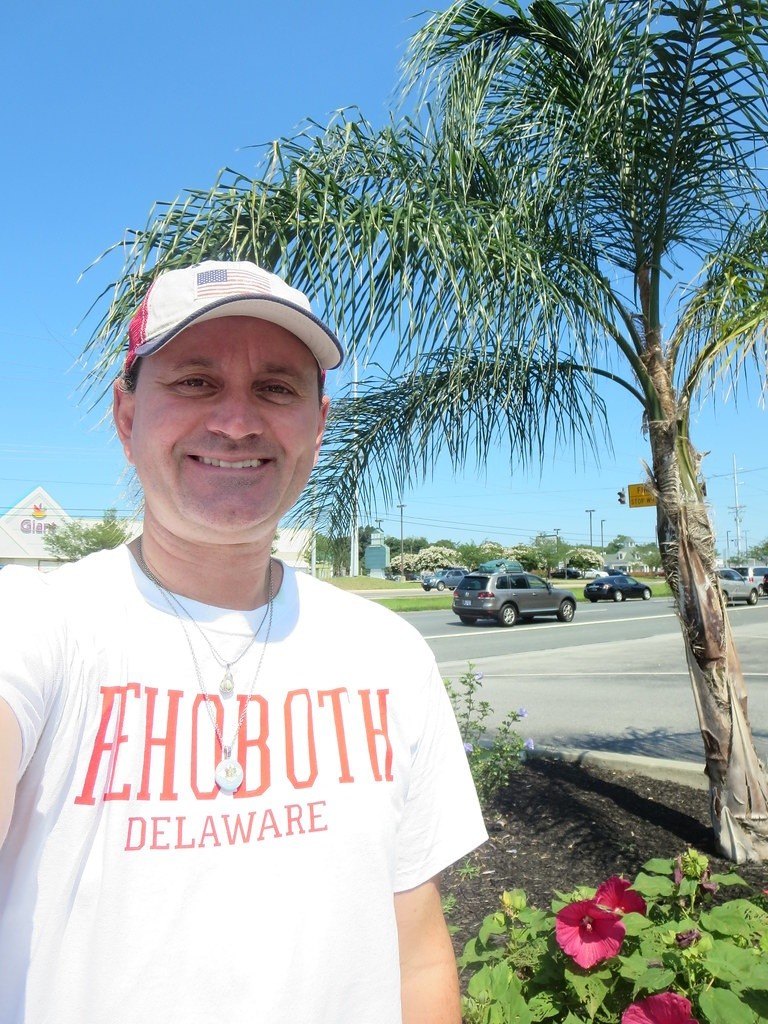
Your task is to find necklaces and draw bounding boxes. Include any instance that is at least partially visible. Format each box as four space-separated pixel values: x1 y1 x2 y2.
136 535 273 792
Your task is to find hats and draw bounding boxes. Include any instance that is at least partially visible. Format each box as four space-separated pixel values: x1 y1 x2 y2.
124 260 345 387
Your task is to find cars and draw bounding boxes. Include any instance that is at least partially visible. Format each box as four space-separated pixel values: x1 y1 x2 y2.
731 566 768 597
551 568 580 579
607 569 631 577
656 569 665 576
578 568 609 579
584 576 652 602
718 569 758 608
762 574 768 594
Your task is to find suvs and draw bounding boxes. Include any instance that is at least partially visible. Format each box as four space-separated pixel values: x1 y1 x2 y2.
421 566 469 591
452 559 577 627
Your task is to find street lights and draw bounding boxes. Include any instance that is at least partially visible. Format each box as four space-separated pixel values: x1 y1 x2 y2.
727 531 731 543
375 519 382 528
726 540 734 566
554 529 560 535
743 530 749 556
586 510 595 546
397 505 406 583
601 520 605 565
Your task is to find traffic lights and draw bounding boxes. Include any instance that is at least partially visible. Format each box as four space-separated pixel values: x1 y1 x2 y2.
618 491 626 504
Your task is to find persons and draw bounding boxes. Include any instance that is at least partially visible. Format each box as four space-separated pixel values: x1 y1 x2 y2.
0 260 490 1024
